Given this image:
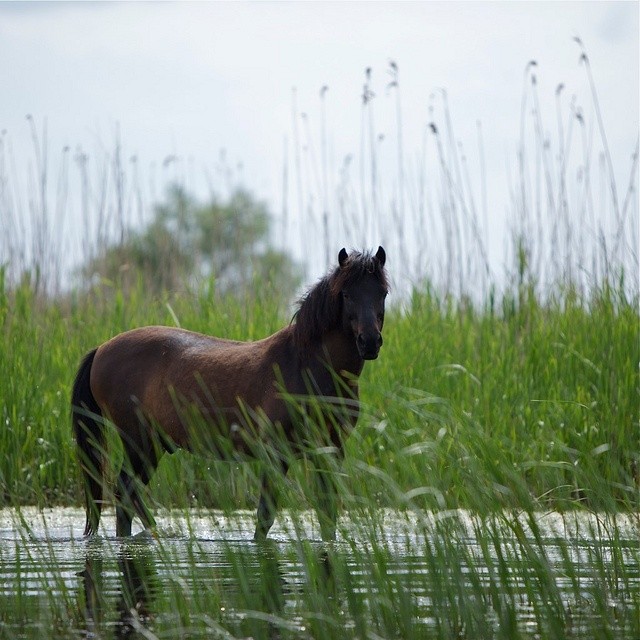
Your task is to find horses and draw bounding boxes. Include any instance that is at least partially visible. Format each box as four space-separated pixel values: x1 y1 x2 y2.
71 245 391 542
